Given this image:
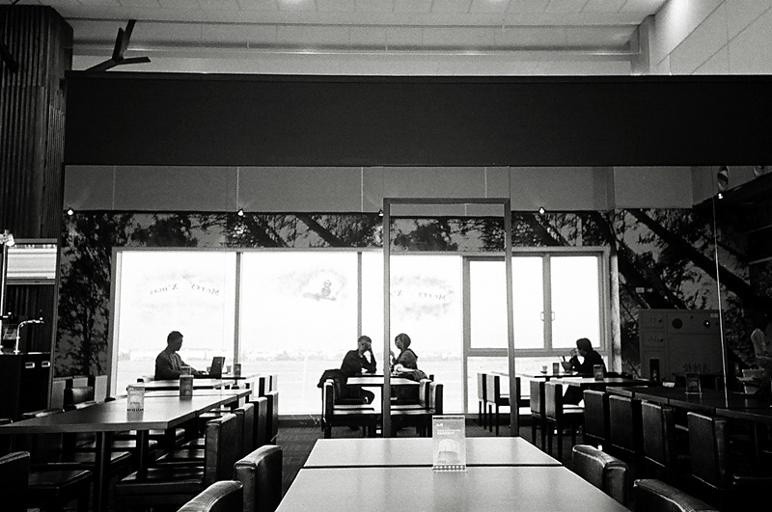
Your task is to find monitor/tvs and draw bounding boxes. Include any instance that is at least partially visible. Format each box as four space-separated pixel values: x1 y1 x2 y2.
5 238 58 285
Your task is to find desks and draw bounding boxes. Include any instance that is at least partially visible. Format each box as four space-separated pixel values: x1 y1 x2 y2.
304 436 564 466
275 464 634 512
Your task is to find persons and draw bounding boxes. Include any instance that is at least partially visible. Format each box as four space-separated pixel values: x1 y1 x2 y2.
154 331 210 442
390 331 418 370
341 335 377 431
563 338 610 406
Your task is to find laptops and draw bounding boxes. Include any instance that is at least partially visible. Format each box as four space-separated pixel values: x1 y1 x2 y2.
201 357 225 376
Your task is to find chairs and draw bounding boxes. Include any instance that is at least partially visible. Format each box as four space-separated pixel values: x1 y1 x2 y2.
1 369 283 510
475 361 772 511
322 366 445 436
